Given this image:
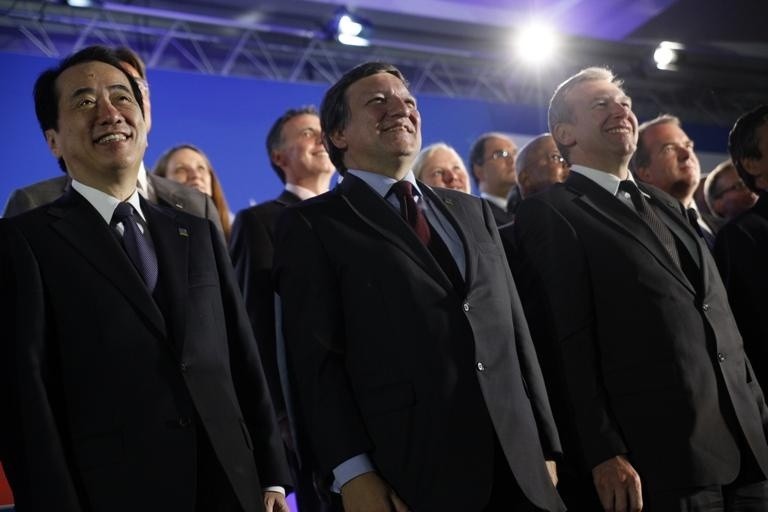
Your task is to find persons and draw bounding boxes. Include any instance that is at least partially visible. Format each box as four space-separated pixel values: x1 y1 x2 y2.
0 47 767 512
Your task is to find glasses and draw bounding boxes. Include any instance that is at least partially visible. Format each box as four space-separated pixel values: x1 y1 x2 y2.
716 178 748 199
481 148 518 164
522 151 570 172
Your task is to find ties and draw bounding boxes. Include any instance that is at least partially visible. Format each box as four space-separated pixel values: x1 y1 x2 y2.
623 178 698 286
689 207 717 251
115 204 163 301
392 178 467 295
